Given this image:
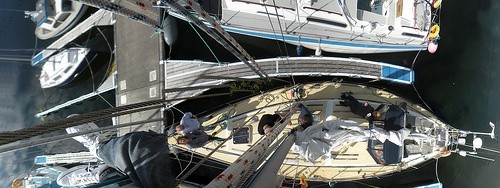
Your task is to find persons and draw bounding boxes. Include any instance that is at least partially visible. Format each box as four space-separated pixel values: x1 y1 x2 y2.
289 102 313 135
338 91 385 124
172 112 217 132
256 114 282 135
55 113 180 188
177 129 226 147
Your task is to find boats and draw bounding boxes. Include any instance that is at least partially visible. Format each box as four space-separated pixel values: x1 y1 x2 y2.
166 0 444 55
39 47 101 89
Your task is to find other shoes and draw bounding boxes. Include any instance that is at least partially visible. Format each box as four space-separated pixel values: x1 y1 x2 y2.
211 127 215 130
209 115 213 119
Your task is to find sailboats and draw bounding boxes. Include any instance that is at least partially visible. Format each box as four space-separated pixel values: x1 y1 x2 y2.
24 0 88 40
60 0 469 187
